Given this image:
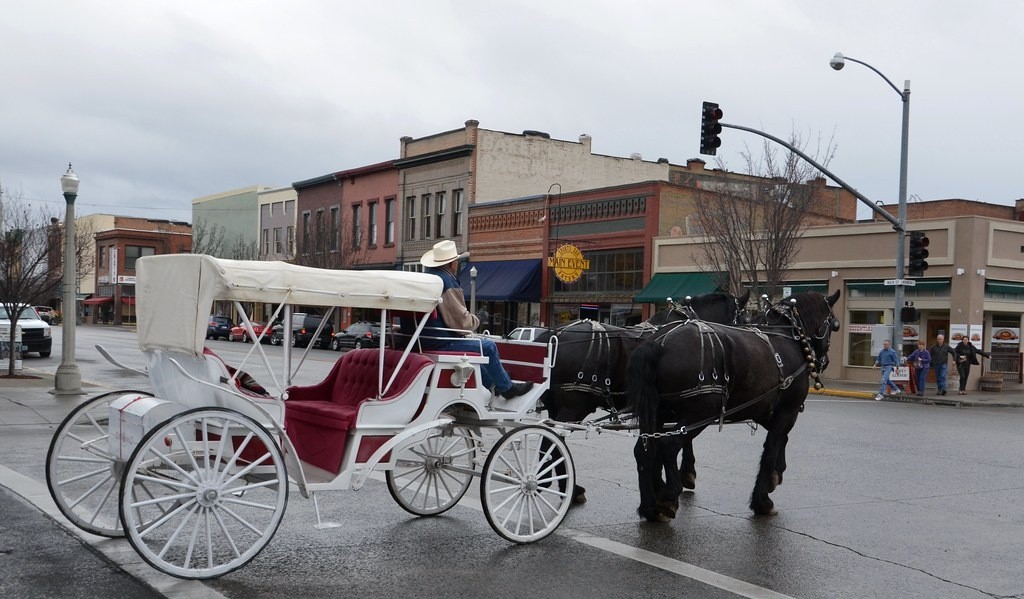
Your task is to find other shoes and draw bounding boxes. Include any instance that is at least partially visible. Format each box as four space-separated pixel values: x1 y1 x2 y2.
958 391 967 395
875 394 883 400
917 391 923 396
490 384 501 397
942 390 947 395
937 390 942 395
501 380 534 400
891 389 901 395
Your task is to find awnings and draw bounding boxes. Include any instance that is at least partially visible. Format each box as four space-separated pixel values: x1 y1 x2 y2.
122 298 135 305
76 294 91 301
83 297 113 304
458 258 542 303
632 271 730 303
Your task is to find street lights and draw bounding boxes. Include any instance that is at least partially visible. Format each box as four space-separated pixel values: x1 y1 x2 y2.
829 51 910 366
46 161 88 396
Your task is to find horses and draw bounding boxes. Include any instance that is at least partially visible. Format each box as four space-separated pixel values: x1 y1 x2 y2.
531 283 754 510
625 286 841 523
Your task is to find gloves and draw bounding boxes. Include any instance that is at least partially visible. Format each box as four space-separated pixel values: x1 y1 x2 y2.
475 306 488 323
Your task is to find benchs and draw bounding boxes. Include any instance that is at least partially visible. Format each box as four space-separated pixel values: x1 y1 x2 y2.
201 306 491 475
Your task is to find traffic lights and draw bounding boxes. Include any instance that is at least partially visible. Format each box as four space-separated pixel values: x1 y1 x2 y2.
901 307 917 322
700 101 723 156
908 231 929 277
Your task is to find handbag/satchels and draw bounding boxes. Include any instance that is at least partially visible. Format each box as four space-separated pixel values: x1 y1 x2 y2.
914 361 923 368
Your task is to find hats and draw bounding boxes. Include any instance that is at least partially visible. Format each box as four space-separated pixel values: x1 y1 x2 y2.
420 240 470 267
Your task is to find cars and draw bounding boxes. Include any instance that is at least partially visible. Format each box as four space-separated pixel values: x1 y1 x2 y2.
331 321 398 352
228 320 273 344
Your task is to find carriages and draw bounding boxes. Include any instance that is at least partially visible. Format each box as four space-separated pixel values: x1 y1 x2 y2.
43 237 845 581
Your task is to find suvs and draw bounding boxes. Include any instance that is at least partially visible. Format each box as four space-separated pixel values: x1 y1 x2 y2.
33 306 53 325
0 302 52 360
205 315 236 340
269 312 335 350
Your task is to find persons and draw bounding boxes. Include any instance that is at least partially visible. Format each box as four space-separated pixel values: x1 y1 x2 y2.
402 240 533 399
873 340 901 401
929 334 957 396
905 340 931 396
954 336 992 394
